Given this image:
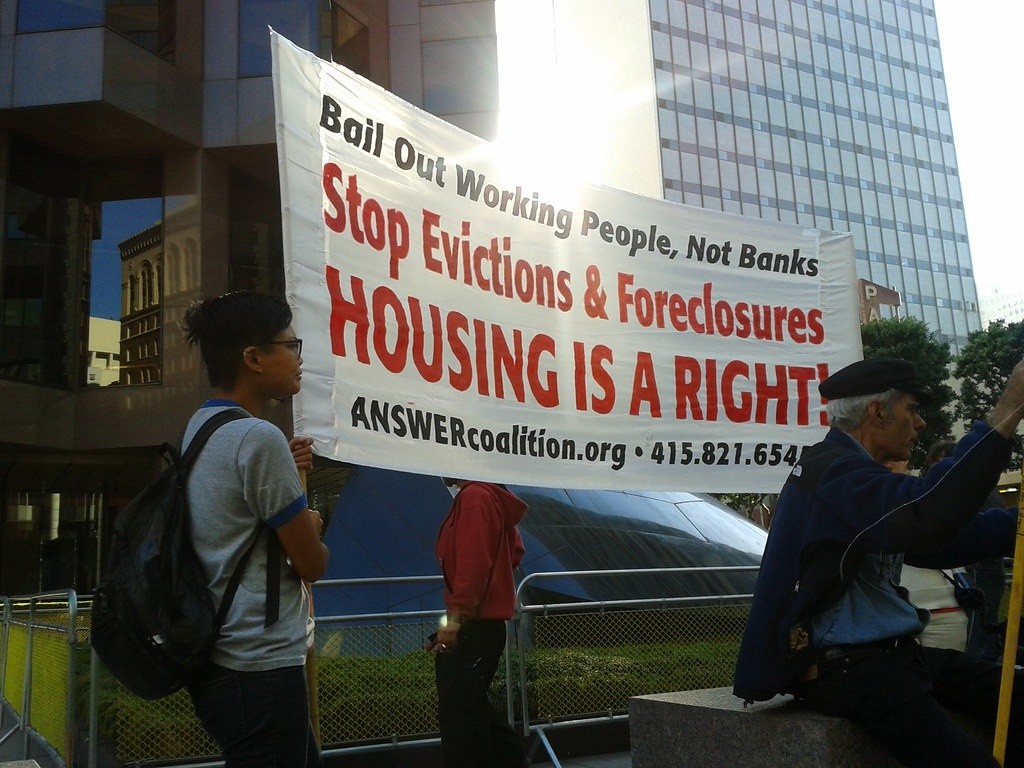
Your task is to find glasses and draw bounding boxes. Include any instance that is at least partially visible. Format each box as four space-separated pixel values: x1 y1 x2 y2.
256 338 304 356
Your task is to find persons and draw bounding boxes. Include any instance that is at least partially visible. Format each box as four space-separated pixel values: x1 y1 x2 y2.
728 353 1024 768
171 292 331 767
423 476 529 767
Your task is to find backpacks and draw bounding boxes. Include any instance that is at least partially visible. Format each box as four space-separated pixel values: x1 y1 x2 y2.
93 409 280 699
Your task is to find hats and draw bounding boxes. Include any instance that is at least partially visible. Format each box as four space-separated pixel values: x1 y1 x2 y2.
818 361 930 409
